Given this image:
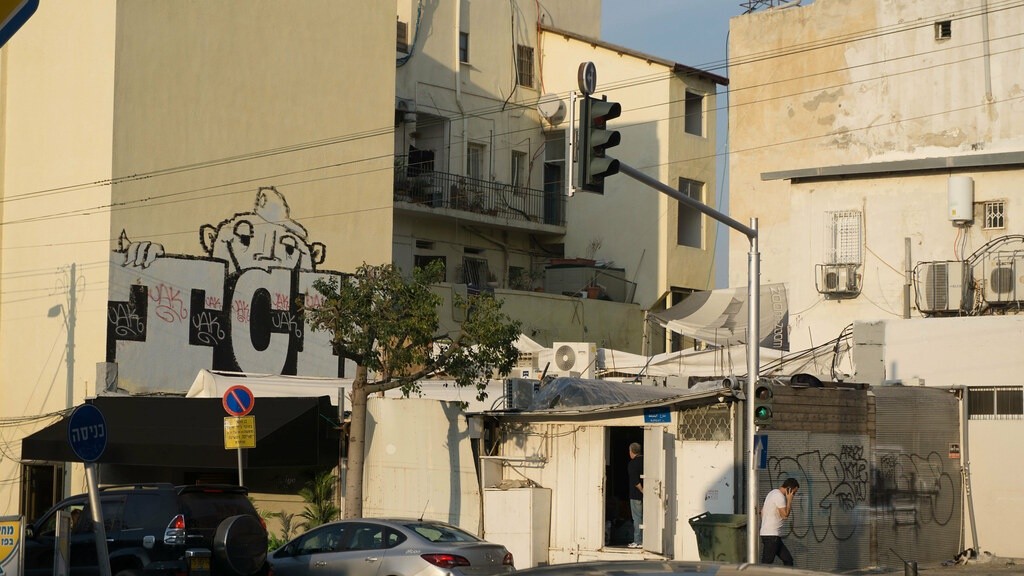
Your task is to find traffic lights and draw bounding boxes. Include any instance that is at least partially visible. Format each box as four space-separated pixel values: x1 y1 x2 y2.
754 377 773 428
578 96 622 193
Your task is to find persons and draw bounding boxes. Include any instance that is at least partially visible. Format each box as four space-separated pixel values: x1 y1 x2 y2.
71 509 82 525
759 478 799 566
627 442 643 547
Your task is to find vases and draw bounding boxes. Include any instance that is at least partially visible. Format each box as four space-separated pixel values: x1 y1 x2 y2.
580 291 589 299
588 287 600 298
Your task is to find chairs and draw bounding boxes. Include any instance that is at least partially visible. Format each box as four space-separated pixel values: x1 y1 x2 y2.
352 529 375 550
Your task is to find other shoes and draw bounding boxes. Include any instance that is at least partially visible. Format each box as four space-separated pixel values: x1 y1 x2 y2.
627 542 642 548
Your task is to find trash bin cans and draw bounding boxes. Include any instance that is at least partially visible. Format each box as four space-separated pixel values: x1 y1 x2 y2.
688 511 748 564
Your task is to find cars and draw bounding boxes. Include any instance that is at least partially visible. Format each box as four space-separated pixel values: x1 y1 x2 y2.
266 517 515 576
25 478 266 576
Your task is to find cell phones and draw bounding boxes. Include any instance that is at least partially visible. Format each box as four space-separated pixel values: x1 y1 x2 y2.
787 487 792 495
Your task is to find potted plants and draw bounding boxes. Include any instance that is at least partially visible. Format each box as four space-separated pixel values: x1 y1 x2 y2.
509 271 544 291
393 156 443 207
451 185 499 216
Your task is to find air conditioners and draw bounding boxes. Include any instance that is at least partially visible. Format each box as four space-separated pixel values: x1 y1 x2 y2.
505 378 541 411
553 341 596 378
984 251 1023 303
822 268 851 291
920 262 970 311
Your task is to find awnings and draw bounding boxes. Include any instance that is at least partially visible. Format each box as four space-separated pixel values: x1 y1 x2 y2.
647 282 788 347
21 396 331 469
